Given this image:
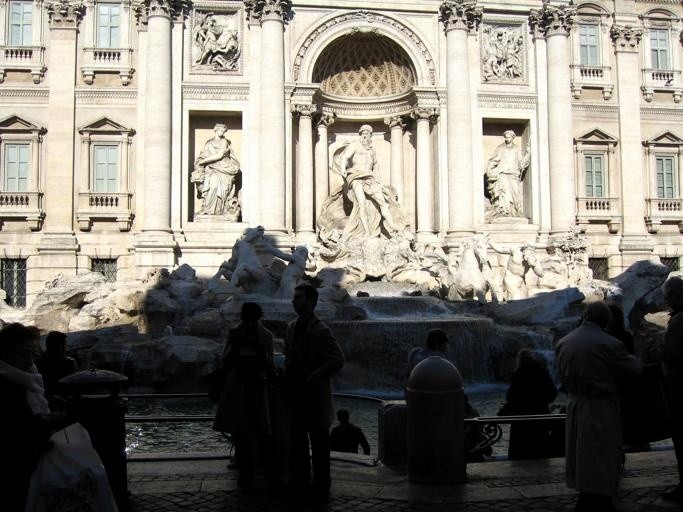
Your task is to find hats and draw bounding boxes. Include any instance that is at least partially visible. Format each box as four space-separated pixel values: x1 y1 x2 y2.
426 330 448 343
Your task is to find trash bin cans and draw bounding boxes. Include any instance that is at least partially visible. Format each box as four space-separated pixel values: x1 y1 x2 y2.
405 355 467 484
58 366 129 510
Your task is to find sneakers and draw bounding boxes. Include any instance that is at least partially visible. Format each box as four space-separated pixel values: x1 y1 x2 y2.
227 463 237 469
661 488 683 498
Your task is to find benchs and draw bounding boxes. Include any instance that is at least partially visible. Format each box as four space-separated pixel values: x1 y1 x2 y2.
467 411 565 457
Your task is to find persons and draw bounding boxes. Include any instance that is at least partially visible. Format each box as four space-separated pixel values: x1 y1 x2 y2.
270 284 346 501
0 322 80 512
340 124 399 236
329 408 371 456
604 304 652 453
552 300 645 512
219 224 309 299
652 277 683 502
486 129 530 217
488 239 544 300
193 123 241 214
195 15 238 69
496 347 559 460
404 328 493 463
483 31 519 81
212 300 274 486
